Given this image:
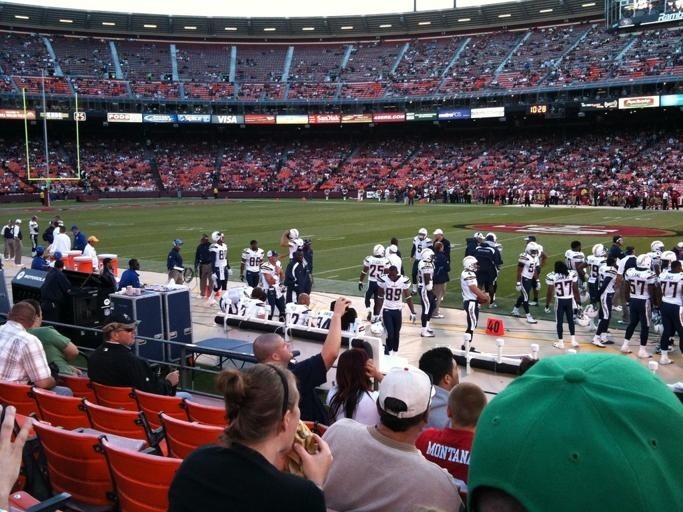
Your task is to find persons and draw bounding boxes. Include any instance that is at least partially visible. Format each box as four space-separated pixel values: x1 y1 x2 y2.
1 404 33 512
416 384 487 512
321 366 464 512
0 215 683 427
168 365 334 511
1 127 683 209
0 24 682 117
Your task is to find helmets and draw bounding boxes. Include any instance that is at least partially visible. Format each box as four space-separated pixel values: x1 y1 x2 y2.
212 230 224 242
173 239 184 247
576 303 598 326
373 227 442 261
288 228 299 239
526 242 539 257
370 320 385 336
636 240 683 269
463 255 481 272
485 231 496 241
15 219 22 224
592 244 609 256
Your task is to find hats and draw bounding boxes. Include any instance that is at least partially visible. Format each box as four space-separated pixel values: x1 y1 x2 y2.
378 365 437 419
524 236 536 240
475 232 485 239
267 250 278 259
103 310 141 330
36 246 64 267
88 236 99 242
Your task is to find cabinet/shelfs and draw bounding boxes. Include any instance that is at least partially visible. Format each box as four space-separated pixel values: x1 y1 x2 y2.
141 288 194 362
108 290 166 365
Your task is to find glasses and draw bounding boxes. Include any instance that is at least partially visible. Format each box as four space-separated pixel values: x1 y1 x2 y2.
423 368 433 412
116 327 133 332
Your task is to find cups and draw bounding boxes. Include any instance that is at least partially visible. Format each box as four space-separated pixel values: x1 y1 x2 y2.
115 286 140 296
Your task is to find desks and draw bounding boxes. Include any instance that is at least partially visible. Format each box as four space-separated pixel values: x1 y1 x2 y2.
194 337 249 372
228 342 301 371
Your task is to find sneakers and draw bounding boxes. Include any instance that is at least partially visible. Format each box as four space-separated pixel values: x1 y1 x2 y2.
611 304 622 311
553 333 674 364
512 301 537 322
421 331 434 337
488 302 497 308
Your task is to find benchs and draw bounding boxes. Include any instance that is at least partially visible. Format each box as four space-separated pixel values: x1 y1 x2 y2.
215 311 382 392
451 349 523 375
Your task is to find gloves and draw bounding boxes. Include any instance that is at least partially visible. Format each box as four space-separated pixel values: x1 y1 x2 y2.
410 312 416 323
544 307 551 312
536 279 541 290
358 282 363 290
516 282 522 292
